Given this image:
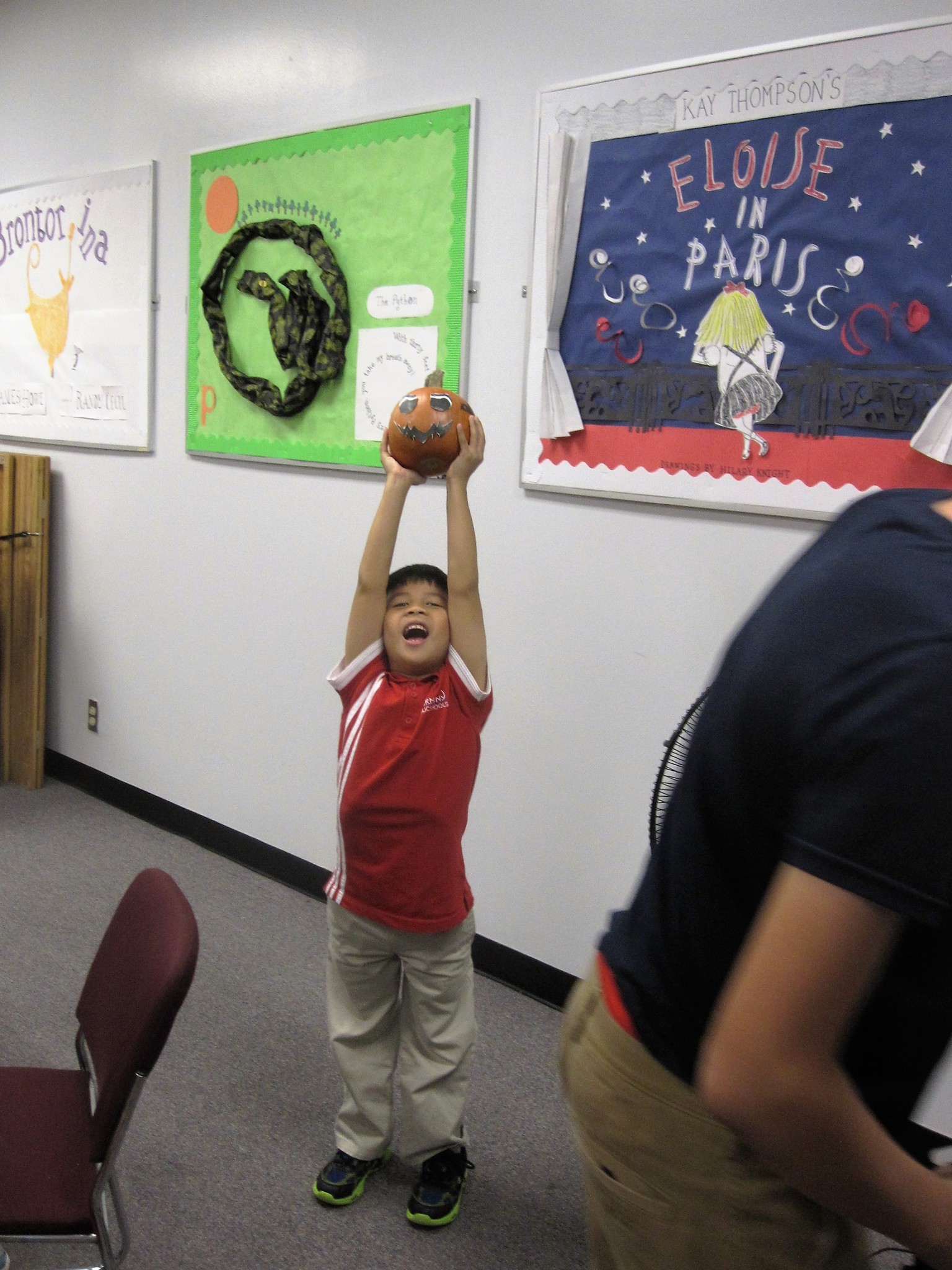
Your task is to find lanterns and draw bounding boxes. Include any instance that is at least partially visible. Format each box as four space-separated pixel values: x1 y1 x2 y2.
387 368 477 480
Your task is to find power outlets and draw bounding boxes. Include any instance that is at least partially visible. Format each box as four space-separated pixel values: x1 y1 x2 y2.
88 698 99 733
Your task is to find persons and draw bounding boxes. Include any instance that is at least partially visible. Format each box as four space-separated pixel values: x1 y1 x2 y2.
548 477 951 1269
305 409 500 1233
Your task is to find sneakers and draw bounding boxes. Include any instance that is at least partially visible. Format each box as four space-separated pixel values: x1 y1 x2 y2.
404 1143 468 1227
312 1144 393 1205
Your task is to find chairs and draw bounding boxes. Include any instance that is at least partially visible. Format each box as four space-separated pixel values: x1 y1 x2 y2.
1 866 206 1270
